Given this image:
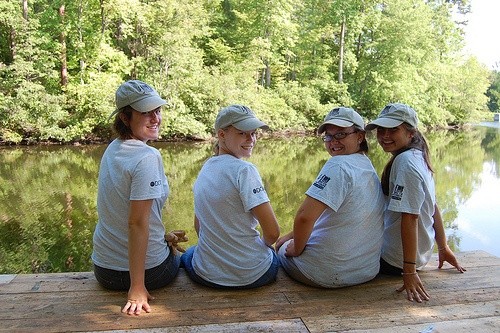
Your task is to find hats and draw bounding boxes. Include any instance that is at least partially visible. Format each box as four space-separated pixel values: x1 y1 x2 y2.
107 80 168 124
366 103 417 130
317 107 364 134
215 105 269 132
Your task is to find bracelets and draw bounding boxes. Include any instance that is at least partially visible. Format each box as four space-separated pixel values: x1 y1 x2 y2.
403 261 416 264
401 272 417 275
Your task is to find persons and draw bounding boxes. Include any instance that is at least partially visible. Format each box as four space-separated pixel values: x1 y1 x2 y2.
275 107 383 289
180 105 279 289
366 103 467 303
91 80 187 316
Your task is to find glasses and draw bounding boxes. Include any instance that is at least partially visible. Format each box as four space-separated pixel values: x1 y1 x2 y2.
322 130 360 142
